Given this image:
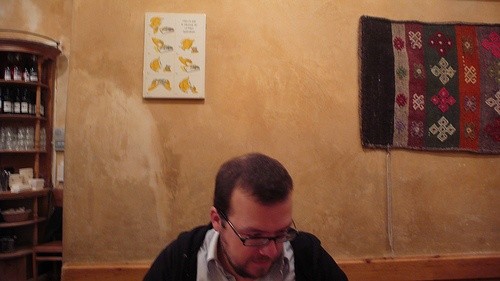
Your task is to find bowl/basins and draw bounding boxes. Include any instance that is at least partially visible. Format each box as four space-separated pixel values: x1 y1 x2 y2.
8 168 44 194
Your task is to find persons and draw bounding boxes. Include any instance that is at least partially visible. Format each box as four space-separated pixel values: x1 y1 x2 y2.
143 151 349 281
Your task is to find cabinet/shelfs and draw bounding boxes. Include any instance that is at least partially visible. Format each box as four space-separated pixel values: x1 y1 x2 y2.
0 28 64 281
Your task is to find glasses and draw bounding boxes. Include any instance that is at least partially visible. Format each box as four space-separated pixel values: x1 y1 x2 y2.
214 204 299 248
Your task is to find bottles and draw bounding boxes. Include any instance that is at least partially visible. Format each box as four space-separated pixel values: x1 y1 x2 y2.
0 127 47 152
0 84 46 116
2 170 8 191
4 55 39 81
